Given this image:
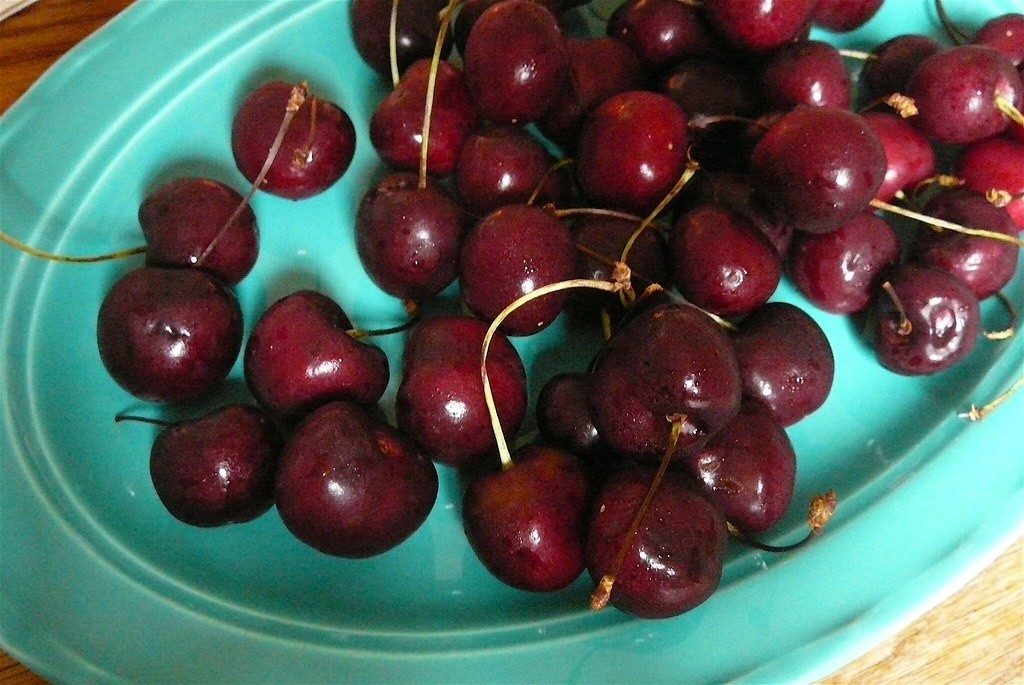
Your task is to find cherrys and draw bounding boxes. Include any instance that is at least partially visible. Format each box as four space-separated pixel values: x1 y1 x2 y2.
0 0 1024 619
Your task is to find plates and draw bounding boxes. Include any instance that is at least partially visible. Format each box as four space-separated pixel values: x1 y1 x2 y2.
0 0 1024 685
